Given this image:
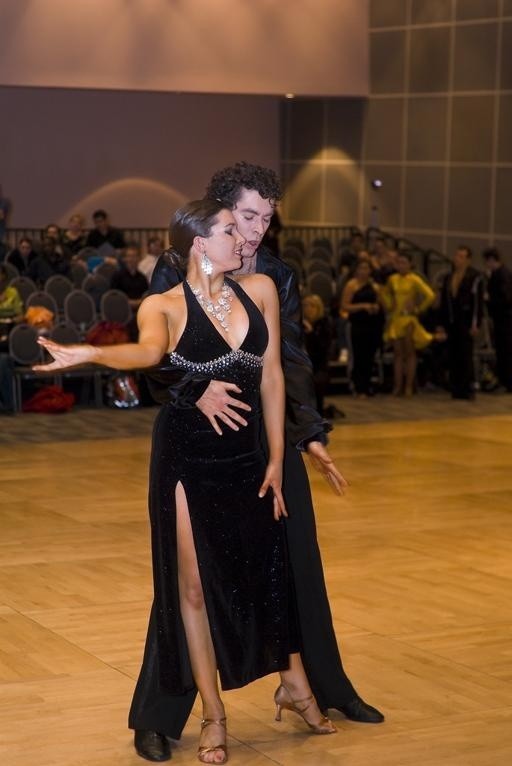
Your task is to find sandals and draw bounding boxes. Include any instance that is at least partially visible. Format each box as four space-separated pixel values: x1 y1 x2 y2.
197 716 229 765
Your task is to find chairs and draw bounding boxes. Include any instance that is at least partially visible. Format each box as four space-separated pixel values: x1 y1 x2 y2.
0 246 133 416
281 237 427 394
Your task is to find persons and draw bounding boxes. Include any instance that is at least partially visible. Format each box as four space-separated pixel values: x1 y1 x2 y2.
8 208 163 301
31 200 339 764
283 228 511 408
126 161 385 761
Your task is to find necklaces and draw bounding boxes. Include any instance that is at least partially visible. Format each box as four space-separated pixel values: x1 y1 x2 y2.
188 280 235 329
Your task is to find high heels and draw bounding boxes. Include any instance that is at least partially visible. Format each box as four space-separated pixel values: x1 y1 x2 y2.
273 683 340 735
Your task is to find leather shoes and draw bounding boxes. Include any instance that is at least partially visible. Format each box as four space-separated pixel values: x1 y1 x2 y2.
131 727 172 762
314 690 386 724
